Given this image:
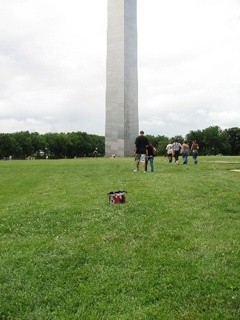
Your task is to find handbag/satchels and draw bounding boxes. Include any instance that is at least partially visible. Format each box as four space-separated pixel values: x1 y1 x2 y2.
152 150 157 156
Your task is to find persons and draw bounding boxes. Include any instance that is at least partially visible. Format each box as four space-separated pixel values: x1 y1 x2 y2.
166 140 189 165
191 140 199 164
134 131 157 173
